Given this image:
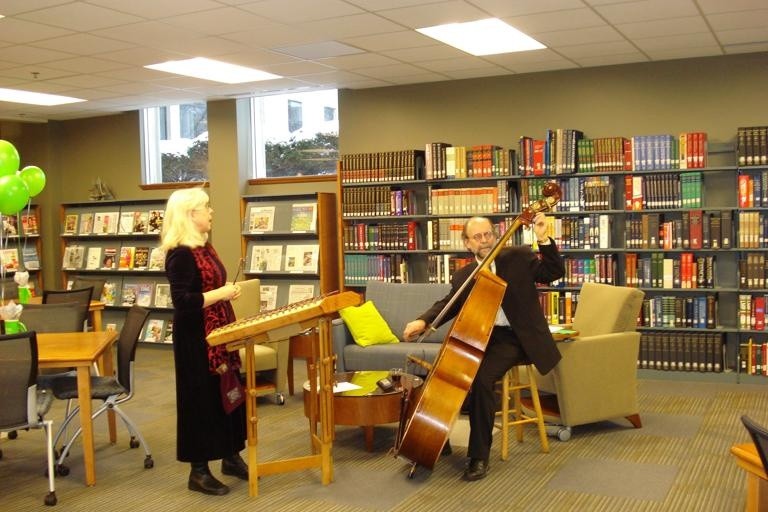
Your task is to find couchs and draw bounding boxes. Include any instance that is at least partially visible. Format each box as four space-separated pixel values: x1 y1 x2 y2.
514 281 645 442
332 281 455 377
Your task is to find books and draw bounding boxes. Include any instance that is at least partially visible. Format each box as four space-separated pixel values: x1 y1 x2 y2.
93 213 118 234
427 217 471 284
88 247 101 271
154 283 172 307
65 215 79 234
166 321 174 342
250 245 283 272
120 211 147 235
548 292 552 324
138 282 152 307
535 252 543 287
65 246 84 268
124 283 136 307
494 217 566 249
120 247 135 271
104 322 117 332
147 319 163 344
289 203 318 236
152 246 163 270
340 148 424 285
566 215 621 325
623 122 768 378
560 296 566 324
2 205 46 299
258 285 278 312
550 281 553 286
426 126 622 212
65 278 73 292
559 254 564 288
553 280 560 289
248 207 275 232
551 292 560 325
149 211 164 233
80 214 93 234
101 280 119 306
538 292 543 314
288 285 315 307
136 245 149 271
286 243 321 275
543 294 548 323
103 248 117 270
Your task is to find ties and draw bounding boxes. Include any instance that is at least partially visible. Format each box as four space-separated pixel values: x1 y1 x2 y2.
476 255 493 274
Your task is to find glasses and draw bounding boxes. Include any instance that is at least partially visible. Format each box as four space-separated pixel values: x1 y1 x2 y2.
192 203 211 211
468 231 496 242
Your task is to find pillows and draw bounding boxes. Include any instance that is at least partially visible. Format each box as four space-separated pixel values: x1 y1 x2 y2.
337 300 400 348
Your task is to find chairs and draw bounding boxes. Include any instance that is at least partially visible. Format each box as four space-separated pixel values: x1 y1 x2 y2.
42 285 95 306
222 277 291 405
20 307 98 458
44 305 155 479
1 330 60 507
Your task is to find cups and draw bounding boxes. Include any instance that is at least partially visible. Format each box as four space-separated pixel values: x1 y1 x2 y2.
18 286 31 305
6 321 26 335
392 369 402 384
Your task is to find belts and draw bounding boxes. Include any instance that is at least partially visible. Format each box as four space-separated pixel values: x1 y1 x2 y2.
497 326 515 333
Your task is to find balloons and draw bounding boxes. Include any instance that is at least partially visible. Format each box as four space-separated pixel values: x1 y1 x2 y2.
1 138 20 178
20 166 47 197
0 176 29 216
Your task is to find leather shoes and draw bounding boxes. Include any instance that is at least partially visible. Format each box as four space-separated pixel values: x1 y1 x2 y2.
441 445 451 456
187 471 229 495
464 457 490 480
222 459 260 482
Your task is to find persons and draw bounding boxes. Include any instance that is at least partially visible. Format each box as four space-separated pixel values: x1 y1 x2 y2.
159 188 259 495
401 211 567 482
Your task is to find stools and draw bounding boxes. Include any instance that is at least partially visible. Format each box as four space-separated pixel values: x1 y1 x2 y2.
492 358 549 461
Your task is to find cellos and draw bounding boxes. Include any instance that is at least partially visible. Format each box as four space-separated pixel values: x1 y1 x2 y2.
390 180 563 481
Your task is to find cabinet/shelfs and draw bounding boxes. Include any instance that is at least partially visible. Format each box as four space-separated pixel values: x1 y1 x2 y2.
238 192 335 358
59 200 171 344
0 203 44 308
336 143 767 386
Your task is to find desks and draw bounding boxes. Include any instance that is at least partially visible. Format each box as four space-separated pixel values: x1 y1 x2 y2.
32 331 115 487
